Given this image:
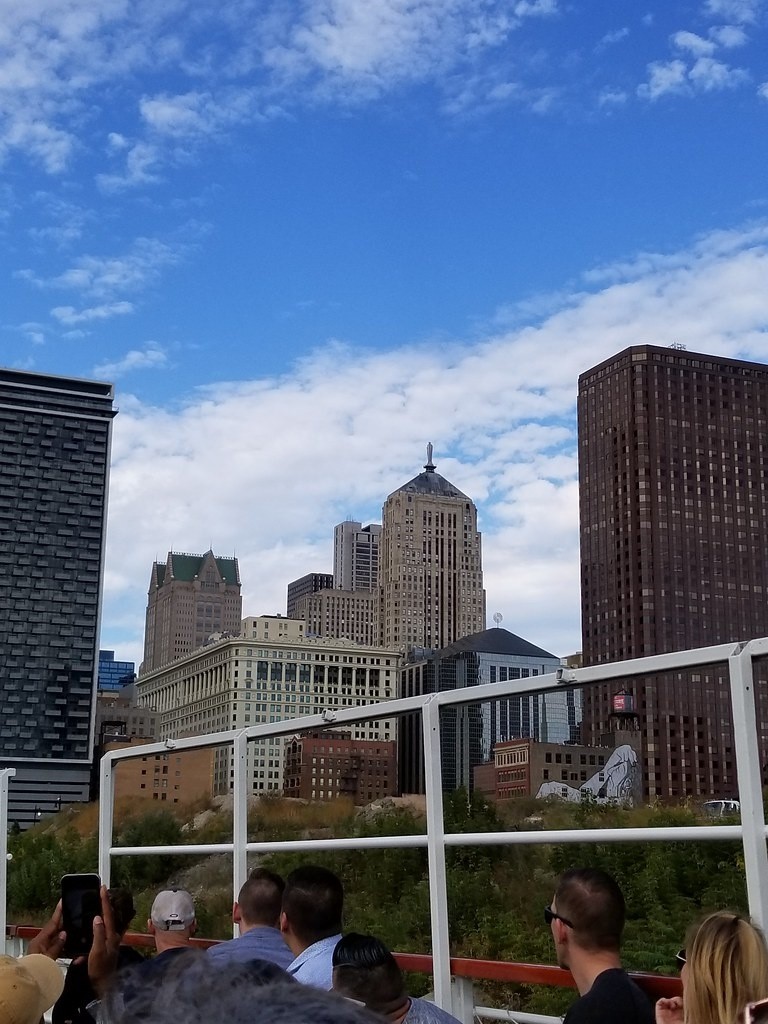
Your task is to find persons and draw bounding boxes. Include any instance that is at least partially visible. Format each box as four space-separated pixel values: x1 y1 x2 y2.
0 861 398 1024
549 867 657 1024
332 933 463 1024
655 910 768 1024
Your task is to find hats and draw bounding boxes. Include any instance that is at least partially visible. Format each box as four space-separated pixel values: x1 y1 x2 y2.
106 887 137 935
151 889 195 931
0 954 66 1024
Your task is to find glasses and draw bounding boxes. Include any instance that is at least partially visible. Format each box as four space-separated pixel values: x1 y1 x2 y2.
544 907 574 928
743 997 768 1024
676 949 687 970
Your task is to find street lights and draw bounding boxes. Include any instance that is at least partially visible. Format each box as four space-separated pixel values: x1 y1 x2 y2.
34 804 42 826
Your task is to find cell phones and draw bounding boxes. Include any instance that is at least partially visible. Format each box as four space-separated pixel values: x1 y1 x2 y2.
60 873 101 958
